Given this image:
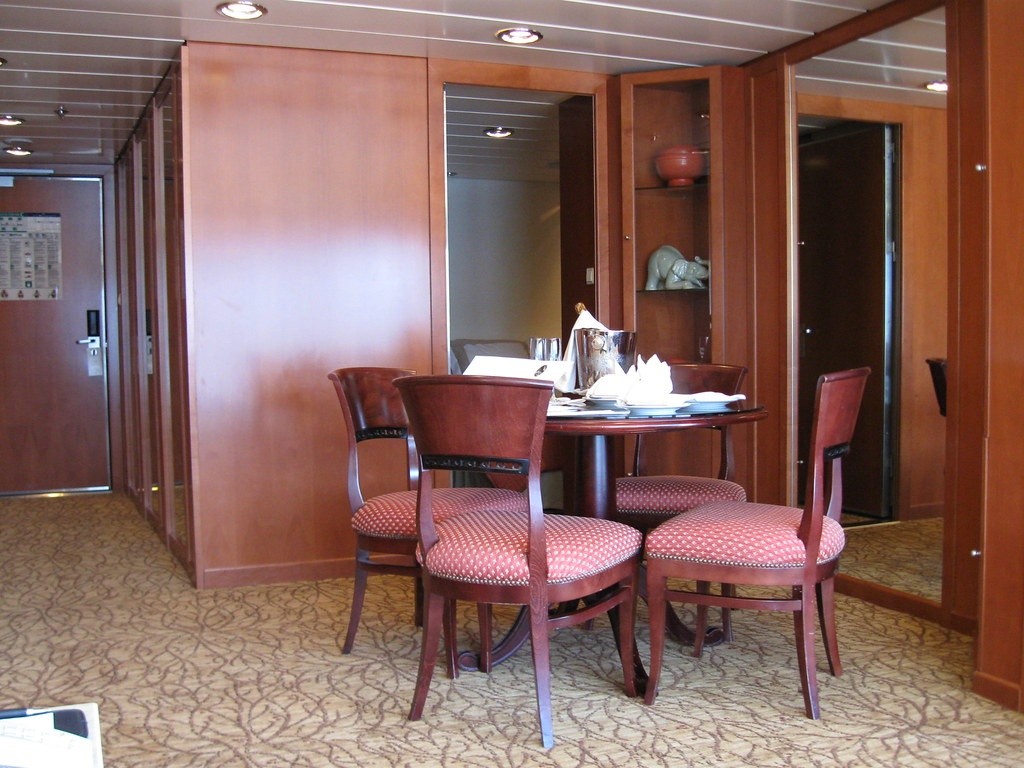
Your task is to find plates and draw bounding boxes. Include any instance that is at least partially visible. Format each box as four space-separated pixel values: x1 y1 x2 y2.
547 393 736 419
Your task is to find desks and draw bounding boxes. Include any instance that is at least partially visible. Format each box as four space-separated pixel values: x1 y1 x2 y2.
454 403 769 698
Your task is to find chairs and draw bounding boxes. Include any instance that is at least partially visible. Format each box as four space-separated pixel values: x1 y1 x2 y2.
329 367 528 679
484 434 579 515
644 366 872 721
391 375 642 749
581 364 747 644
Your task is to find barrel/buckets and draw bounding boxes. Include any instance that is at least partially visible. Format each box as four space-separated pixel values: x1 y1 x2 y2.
574 329 638 391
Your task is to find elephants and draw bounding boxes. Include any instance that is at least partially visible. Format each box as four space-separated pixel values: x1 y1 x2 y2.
645 245 709 290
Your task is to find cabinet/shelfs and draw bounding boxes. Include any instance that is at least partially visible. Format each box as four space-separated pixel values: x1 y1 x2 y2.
618 63 746 480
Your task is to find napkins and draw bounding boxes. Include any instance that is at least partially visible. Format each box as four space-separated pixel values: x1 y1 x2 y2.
549 312 746 408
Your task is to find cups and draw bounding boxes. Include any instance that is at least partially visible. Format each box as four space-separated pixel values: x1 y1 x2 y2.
530 337 562 362
